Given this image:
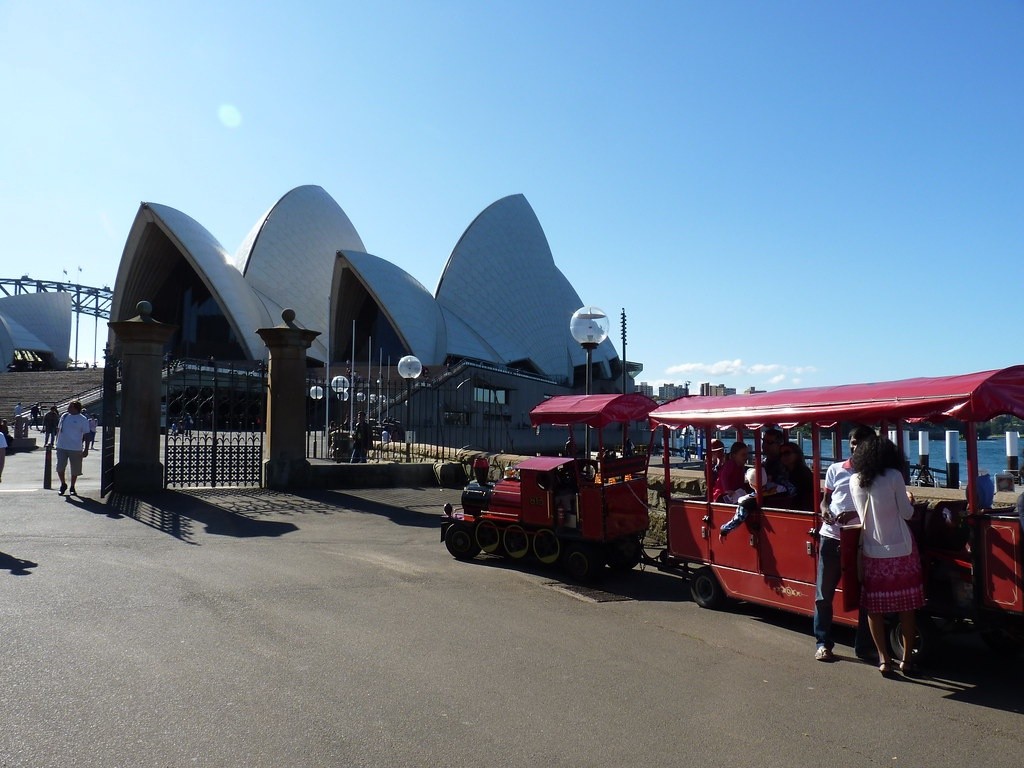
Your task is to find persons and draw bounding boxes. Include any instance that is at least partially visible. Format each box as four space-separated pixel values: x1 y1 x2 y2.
565 437 572 458
15 403 23 416
704 430 814 544
0 419 10 482
42 406 59 448
351 413 373 463
56 401 89 494
328 416 350 451
598 449 617 461
813 425 878 660
171 413 193 436
30 402 40 431
82 408 98 449
626 437 635 456
382 423 402 443
849 437 925 674
683 449 690 463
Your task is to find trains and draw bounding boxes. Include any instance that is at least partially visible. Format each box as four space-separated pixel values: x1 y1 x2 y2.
437 361 1024 679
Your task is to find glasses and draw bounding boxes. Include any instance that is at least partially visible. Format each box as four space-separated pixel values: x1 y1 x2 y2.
762 438 780 445
778 450 794 459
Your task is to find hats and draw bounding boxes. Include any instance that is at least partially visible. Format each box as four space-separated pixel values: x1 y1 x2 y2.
745 468 767 486
711 438 723 452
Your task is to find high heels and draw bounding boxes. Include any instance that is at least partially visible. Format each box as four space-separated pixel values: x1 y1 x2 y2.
899 661 917 675
879 664 893 678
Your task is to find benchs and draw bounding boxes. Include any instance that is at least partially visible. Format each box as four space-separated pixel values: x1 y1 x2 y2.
579 455 649 542
724 485 971 601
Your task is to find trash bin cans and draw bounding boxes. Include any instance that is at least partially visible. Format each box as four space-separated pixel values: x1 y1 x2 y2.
994 473 1015 492
330 430 351 463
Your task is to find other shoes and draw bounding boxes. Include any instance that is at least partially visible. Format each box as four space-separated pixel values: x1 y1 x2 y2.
70 488 75 495
859 650 881 662
58 483 67 495
815 647 831 659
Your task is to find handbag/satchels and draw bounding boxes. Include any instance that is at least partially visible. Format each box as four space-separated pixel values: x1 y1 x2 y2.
856 547 865 583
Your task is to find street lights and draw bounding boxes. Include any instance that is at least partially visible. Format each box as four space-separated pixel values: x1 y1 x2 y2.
309 385 324 433
568 305 610 478
397 355 423 462
330 376 387 441
